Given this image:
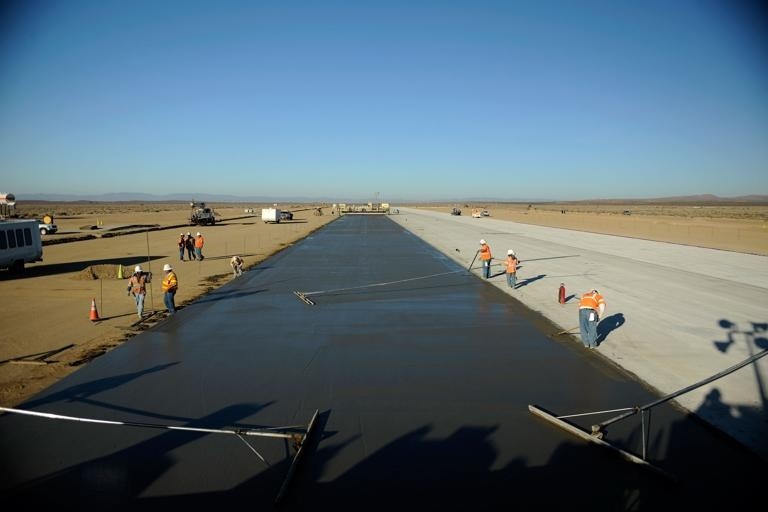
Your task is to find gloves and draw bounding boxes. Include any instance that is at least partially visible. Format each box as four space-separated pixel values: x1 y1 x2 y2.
127 291 131 296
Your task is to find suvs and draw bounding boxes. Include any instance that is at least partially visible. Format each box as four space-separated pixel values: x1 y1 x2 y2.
188 206 219 227
279 210 292 220
0 215 44 276
32 218 59 236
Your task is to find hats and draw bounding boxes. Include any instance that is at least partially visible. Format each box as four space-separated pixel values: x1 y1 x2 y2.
507 249 514 256
479 239 487 245
163 264 173 272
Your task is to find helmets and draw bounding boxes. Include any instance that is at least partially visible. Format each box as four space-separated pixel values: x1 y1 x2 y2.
180 231 201 237
134 265 144 273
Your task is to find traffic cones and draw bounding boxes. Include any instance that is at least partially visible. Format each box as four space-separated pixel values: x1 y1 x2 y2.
116 263 125 280
86 295 100 323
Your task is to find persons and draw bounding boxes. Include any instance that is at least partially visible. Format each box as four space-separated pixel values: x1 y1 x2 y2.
502 250 520 289
578 290 606 349
161 264 178 314
127 265 152 321
478 239 492 279
195 232 204 261
229 255 244 278
178 233 185 262
184 232 196 260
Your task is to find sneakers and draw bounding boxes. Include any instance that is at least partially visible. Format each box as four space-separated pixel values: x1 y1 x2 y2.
481 275 491 280
583 341 599 350
136 312 144 320
507 284 516 289
179 255 204 262
163 309 175 316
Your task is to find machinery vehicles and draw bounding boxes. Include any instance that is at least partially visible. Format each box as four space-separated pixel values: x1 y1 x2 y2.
446 204 493 219
